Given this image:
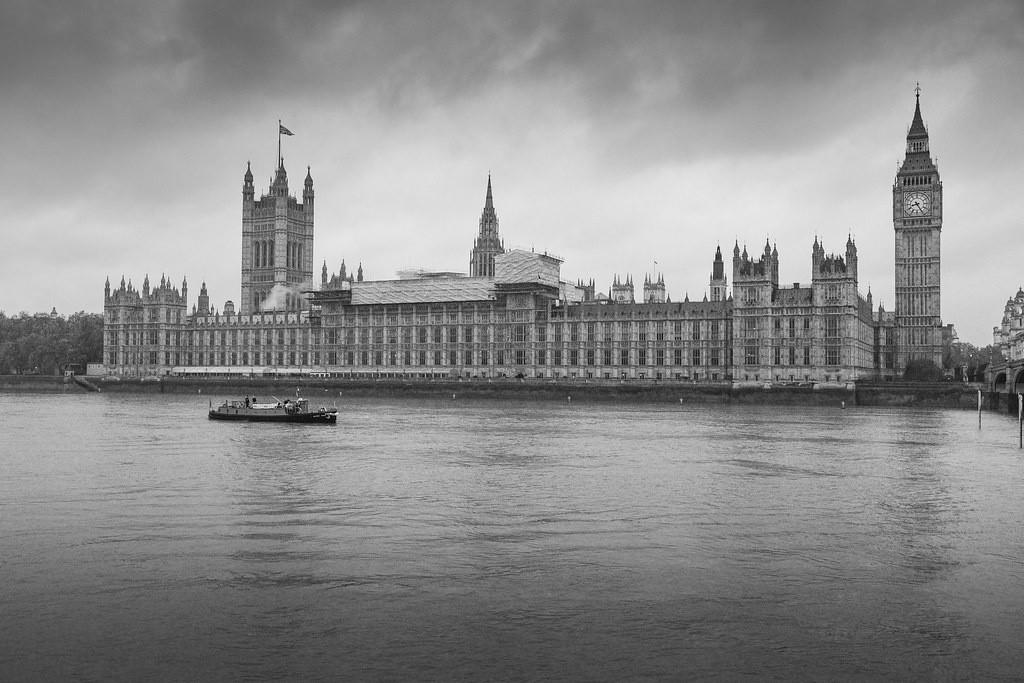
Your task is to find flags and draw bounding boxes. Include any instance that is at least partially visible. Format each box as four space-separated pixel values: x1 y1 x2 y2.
280 124 295 136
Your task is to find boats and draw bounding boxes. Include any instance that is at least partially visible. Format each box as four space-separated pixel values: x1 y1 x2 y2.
208 387 339 423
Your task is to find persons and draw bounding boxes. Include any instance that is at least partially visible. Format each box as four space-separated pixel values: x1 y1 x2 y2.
252 396 257 403
245 394 249 408
